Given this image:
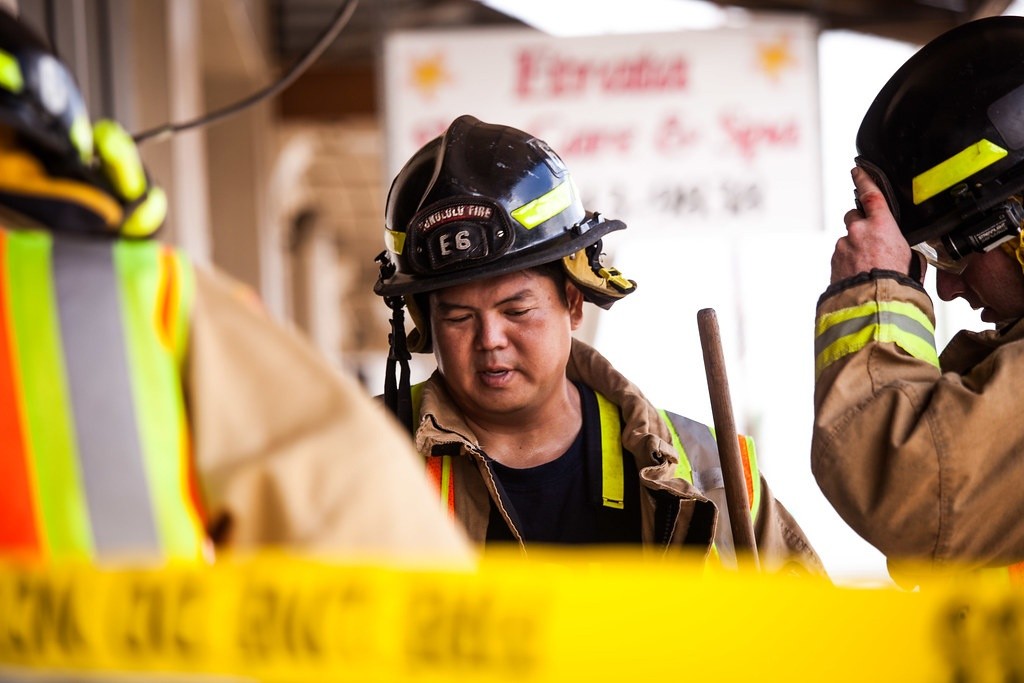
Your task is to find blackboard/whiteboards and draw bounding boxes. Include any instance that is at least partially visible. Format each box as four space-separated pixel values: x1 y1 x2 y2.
370 17 853 571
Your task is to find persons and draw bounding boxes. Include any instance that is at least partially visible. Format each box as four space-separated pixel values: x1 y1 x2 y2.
370 115 833 587
0 10 476 571
811 14 1024 590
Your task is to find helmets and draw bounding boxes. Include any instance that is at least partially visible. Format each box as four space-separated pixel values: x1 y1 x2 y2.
855 16 1024 247
374 115 627 297
0 0 167 238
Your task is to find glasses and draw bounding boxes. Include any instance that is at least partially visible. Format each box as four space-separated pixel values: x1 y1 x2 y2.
915 197 1024 274
